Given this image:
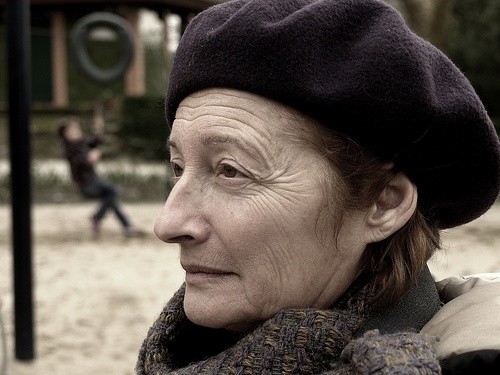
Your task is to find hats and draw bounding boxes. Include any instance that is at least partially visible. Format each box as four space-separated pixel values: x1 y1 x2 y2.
165 1 500 230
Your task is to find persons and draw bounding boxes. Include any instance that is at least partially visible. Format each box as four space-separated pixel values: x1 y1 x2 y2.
134 0 499 375
59 115 147 238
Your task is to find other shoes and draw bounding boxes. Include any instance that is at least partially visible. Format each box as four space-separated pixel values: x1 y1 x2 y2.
123 226 147 239
89 217 103 241
